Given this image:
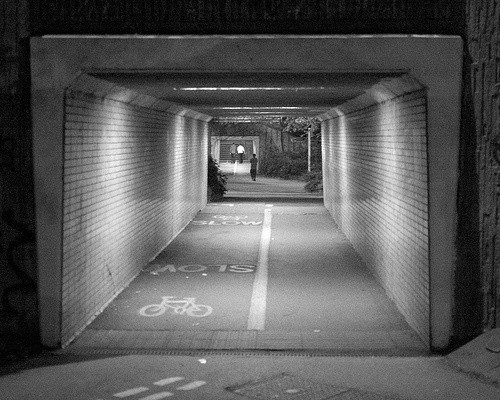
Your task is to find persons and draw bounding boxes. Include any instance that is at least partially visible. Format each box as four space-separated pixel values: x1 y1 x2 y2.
229 143 237 163
249 154 258 181
237 144 246 164
